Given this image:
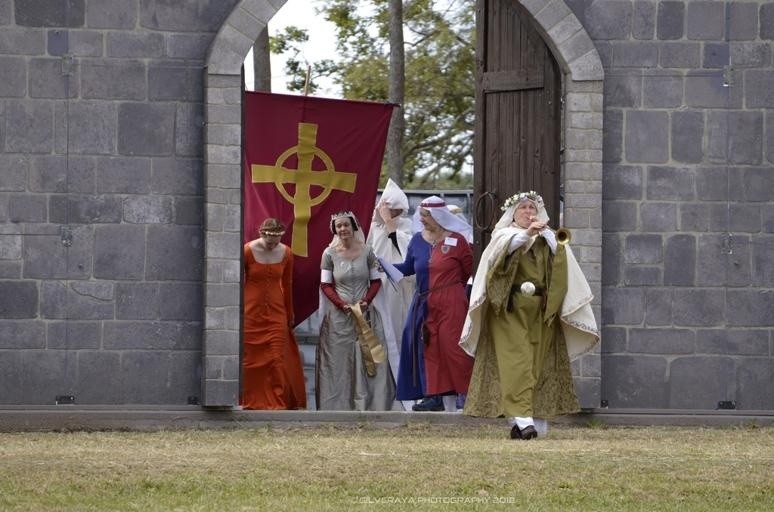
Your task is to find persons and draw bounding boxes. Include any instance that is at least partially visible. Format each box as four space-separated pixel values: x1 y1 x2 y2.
375 196 476 412
457 190 600 440
446 203 466 409
244 217 307 410
314 210 405 411
366 176 424 412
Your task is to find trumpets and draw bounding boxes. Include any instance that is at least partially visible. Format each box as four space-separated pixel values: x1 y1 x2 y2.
525 216 570 246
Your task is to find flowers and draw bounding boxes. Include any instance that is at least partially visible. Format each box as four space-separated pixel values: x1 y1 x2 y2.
499 189 544 212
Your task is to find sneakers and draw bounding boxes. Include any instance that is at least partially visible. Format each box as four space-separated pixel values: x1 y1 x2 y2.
521 425 537 439
510 424 521 439
412 395 446 410
